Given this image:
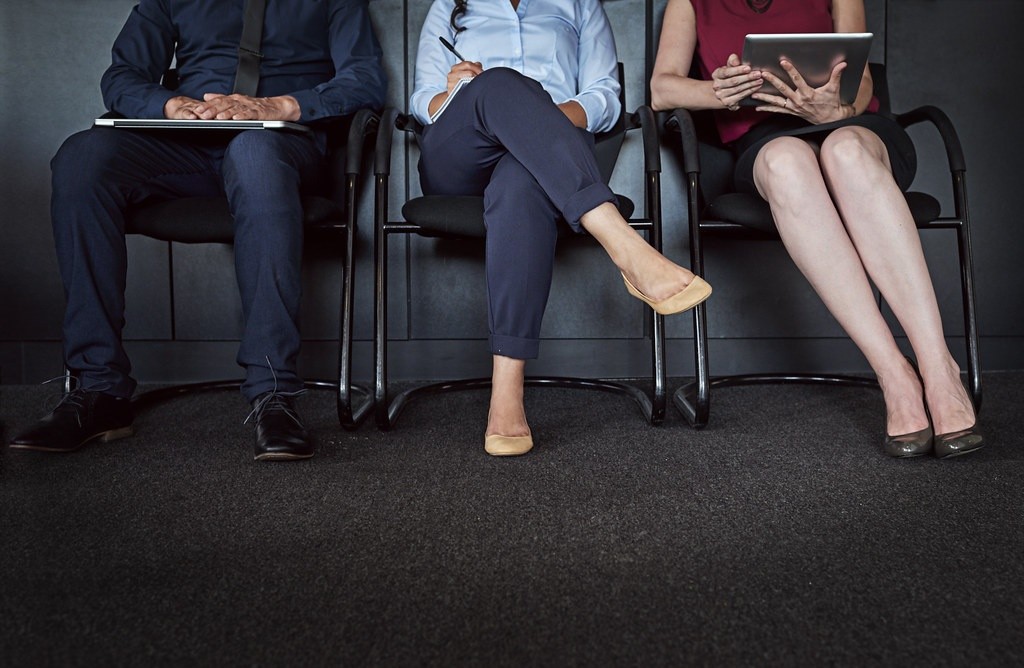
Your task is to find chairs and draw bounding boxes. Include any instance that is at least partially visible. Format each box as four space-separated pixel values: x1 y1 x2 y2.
59 5 987 429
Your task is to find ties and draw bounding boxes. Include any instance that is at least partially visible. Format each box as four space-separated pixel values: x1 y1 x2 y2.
231 0 266 97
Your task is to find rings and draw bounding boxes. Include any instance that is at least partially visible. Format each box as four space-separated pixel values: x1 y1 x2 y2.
784 98 787 108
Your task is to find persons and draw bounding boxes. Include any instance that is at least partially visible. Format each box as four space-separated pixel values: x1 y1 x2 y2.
650 1 985 461
410 0 714 457
7 0 387 461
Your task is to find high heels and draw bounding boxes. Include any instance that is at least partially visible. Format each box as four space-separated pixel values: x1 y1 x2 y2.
924 376 984 459
884 355 934 458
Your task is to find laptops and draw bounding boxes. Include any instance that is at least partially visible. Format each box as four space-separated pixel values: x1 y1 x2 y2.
95 118 309 134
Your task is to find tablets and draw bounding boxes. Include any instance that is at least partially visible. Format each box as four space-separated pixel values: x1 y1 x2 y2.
738 33 873 108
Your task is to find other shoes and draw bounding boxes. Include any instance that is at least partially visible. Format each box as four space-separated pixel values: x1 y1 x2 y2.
484 406 533 457
619 268 714 316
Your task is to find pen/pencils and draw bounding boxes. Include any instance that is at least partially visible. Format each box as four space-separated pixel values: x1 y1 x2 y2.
438 34 469 63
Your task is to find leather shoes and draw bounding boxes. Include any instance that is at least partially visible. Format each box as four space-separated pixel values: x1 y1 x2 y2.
251 391 317 461
10 387 136 452
